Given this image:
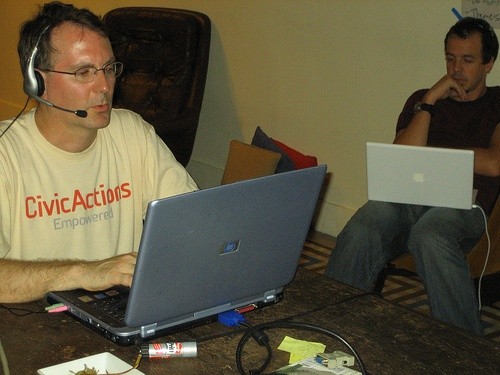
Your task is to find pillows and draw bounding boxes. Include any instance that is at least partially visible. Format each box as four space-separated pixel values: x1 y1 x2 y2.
221 126 317 186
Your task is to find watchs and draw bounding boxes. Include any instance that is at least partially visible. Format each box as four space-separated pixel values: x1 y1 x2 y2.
414 101 438 116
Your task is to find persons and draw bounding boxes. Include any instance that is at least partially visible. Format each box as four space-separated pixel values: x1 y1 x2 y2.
0 0 201 305
324 15 500 337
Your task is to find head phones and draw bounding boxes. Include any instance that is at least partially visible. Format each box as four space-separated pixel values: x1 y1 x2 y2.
23 23 51 98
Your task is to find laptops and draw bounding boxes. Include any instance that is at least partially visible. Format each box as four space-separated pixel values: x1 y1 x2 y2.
366 142 478 210
46 164 327 346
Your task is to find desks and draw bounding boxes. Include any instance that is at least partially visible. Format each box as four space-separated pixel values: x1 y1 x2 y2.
0 267 500 375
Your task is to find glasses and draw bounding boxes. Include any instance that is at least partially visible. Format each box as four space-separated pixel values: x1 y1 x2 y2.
37 62 123 83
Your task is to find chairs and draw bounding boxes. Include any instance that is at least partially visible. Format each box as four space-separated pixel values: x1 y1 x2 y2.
374 193 500 293
102 7 211 168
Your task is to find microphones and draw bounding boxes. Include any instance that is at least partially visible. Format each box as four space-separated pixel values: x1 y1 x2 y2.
33 96 88 118
